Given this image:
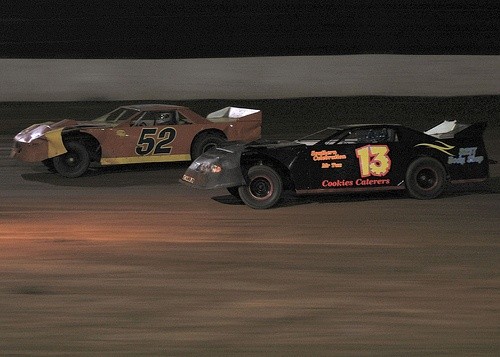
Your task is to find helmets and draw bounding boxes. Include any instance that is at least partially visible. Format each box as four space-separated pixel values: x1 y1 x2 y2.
161 113 172 122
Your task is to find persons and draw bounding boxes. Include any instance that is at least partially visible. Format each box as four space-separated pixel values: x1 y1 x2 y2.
367 129 385 143
156 112 173 125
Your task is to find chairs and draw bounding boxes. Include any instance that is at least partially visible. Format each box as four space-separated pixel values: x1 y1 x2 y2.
387 128 395 143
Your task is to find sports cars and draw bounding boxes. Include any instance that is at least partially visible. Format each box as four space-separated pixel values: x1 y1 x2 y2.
179 120 489 210
9 104 262 177
